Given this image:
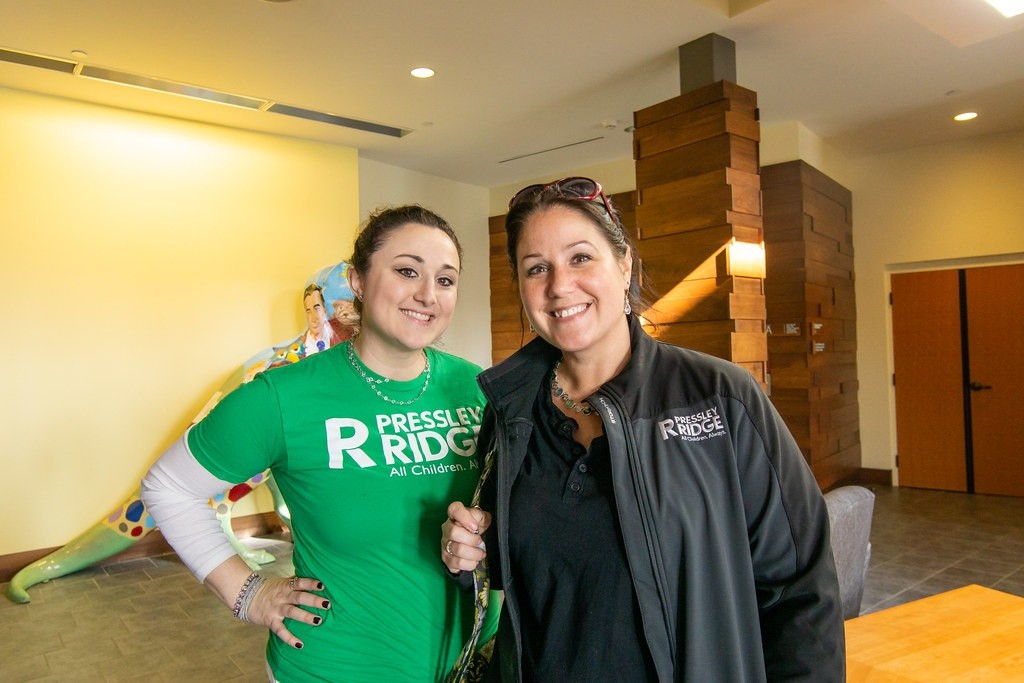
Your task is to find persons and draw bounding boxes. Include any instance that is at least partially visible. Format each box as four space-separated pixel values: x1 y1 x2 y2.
441 177 846 683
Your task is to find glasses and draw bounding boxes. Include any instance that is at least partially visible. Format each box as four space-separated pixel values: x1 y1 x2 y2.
509 176 621 229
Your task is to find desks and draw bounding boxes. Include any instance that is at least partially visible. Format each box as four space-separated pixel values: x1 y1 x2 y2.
844 583 1024 683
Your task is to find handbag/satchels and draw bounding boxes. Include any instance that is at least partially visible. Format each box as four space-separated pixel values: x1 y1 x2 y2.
444 631 497 683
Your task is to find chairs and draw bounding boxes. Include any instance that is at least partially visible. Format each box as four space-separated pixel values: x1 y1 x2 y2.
824 485 876 623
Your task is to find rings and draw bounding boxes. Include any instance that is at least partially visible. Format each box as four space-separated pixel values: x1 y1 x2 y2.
141 206 501 683
445 540 453 555
288 576 298 591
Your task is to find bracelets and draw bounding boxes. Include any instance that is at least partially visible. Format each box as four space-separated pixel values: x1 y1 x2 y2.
232 571 266 622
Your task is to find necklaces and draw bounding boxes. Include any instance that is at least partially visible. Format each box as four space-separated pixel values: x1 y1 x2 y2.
550 356 603 416
347 333 430 405
